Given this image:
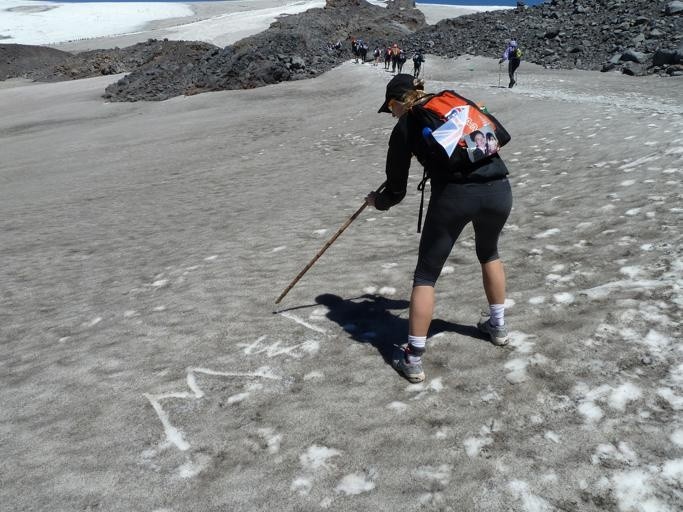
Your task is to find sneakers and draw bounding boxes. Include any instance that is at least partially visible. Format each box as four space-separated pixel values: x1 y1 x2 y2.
508 79 515 88
390 347 425 382
477 317 509 345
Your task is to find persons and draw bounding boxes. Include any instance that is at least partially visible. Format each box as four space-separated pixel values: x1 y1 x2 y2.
484 133 499 155
469 130 488 159
412 51 424 79
372 46 381 67
498 41 521 88
397 48 405 74
351 40 367 65
364 72 515 385
389 43 399 74
383 47 390 70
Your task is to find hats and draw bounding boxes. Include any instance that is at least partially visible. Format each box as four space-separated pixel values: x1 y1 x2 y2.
377 74 424 114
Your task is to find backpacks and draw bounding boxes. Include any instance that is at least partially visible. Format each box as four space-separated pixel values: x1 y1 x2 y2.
411 89 511 170
514 48 521 58
